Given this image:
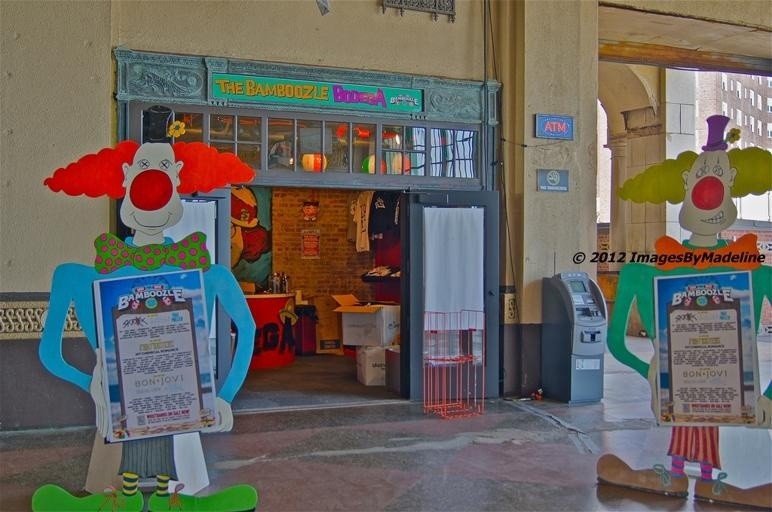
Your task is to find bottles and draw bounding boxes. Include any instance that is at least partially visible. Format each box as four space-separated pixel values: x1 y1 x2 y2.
269 272 289 294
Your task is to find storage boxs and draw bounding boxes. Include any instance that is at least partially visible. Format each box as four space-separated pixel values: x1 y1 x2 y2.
311 292 401 395
